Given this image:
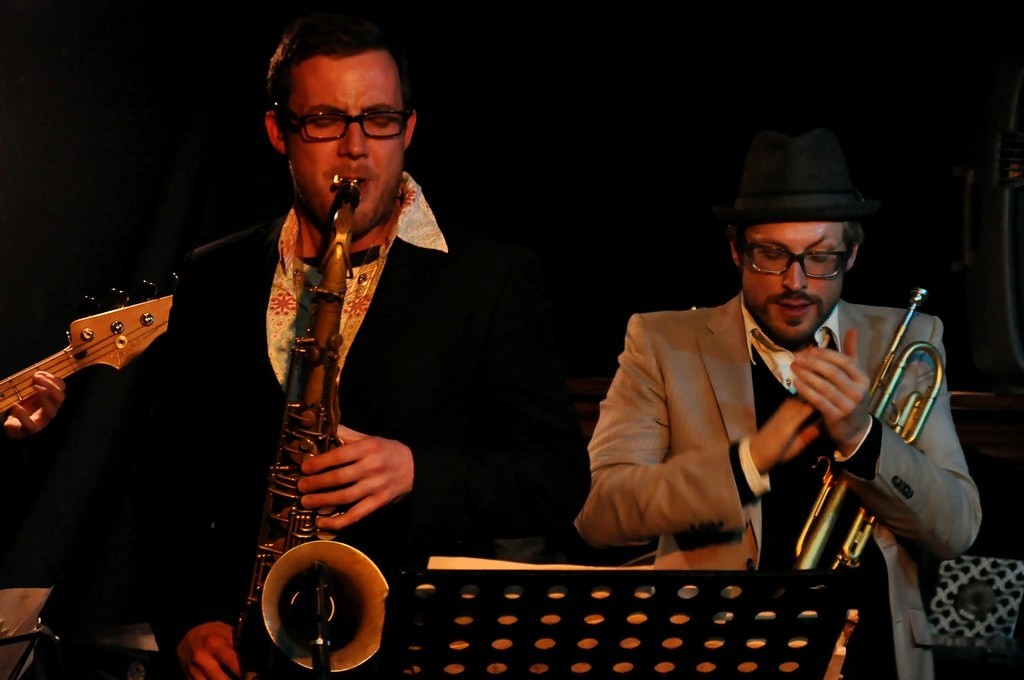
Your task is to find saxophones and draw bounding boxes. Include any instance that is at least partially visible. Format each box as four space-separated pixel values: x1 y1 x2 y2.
230 181 392 679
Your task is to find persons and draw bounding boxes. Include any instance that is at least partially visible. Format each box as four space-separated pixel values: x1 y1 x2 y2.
1 372 67 439
574 131 982 680
107 10 590 680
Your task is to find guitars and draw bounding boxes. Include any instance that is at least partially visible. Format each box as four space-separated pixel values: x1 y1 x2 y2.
1 273 180 417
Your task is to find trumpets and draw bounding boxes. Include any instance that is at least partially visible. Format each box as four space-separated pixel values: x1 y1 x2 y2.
792 283 949 570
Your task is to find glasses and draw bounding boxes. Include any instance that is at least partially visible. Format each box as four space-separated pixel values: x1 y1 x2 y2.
272 103 411 142
747 243 853 279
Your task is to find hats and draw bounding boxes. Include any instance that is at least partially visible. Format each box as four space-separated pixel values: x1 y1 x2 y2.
722 127 870 221
